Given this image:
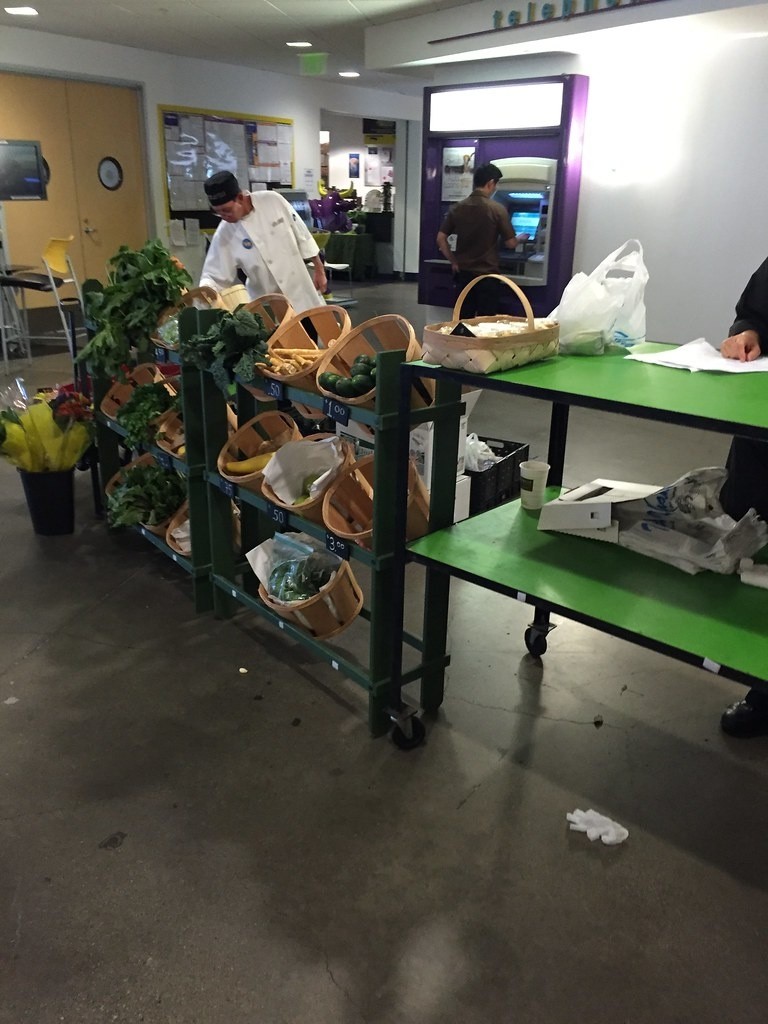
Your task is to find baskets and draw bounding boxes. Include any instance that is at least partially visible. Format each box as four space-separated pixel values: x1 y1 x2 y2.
420 273 560 374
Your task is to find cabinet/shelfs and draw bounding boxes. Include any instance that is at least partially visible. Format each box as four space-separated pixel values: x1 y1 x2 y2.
80 277 466 725
409 320 768 775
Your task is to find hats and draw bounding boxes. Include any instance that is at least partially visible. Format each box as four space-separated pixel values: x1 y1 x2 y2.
204 170 241 206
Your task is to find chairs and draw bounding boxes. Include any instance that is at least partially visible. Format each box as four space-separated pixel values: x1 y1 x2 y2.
11 234 93 364
310 231 353 301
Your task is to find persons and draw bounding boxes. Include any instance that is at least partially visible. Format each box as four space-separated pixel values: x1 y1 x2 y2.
197 172 328 346
437 162 530 319
716 259 768 738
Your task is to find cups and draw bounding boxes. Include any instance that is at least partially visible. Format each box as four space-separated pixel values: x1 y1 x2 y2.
519 461 550 510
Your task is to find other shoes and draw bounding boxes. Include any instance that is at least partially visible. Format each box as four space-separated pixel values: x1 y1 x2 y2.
720 700 768 737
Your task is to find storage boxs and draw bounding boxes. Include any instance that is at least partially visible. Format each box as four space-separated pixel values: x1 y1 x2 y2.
337 390 529 525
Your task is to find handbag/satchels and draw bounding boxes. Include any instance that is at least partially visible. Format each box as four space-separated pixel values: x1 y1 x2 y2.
547 239 649 354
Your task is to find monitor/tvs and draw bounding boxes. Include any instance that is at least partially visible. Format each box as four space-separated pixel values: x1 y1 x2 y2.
0 139 47 201
509 210 540 243
